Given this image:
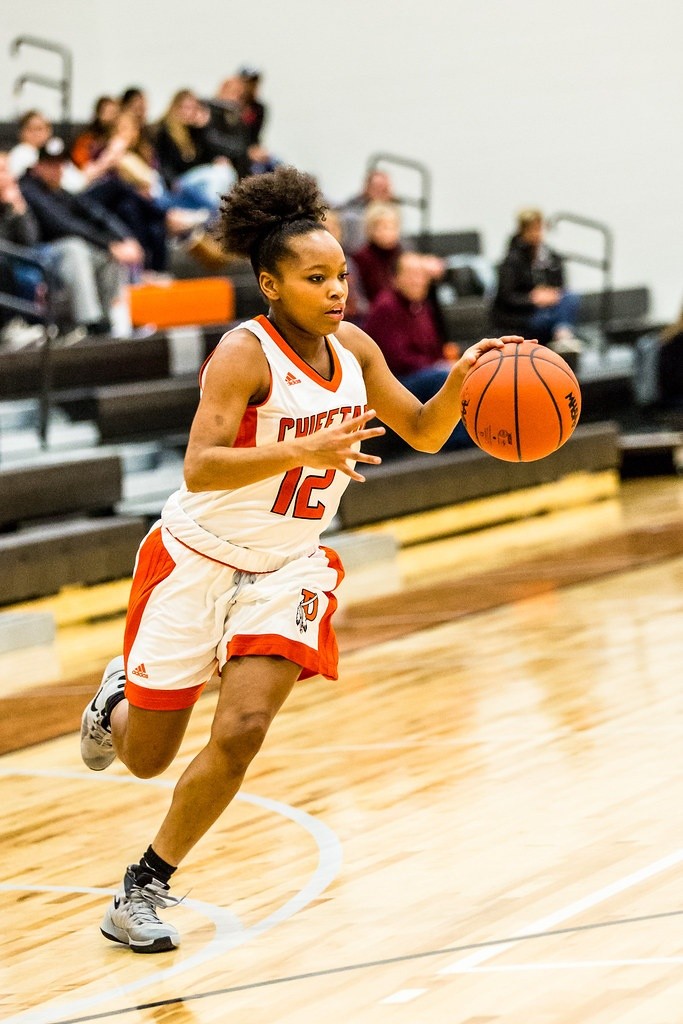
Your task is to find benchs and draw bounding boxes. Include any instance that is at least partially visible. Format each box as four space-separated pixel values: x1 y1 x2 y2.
0 119 672 610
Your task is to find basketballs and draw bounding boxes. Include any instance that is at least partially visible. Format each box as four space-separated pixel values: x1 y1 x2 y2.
457 339 582 466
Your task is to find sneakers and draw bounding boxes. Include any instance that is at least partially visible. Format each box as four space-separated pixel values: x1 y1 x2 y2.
100 865 190 953
80 656 131 771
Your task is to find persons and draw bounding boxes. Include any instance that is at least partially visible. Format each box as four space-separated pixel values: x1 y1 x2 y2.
357 207 461 358
651 310 683 467
0 70 281 353
492 208 586 353
364 252 473 452
319 211 365 322
79 172 538 954
356 171 400 206
